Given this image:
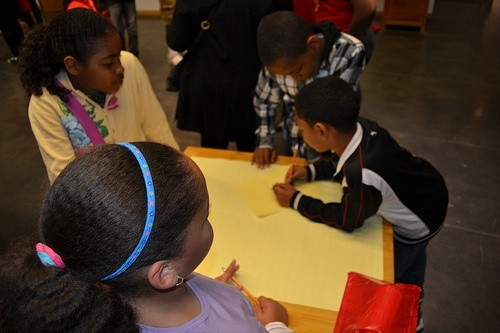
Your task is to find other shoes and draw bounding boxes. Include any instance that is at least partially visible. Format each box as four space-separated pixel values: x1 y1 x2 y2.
167 48 185 66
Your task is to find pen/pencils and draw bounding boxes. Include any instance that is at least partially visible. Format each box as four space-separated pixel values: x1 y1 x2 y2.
221 267 263 305
289 143 299 179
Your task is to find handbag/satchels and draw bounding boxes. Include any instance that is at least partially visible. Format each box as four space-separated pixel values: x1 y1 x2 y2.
166 57 193 91
333 272 421 333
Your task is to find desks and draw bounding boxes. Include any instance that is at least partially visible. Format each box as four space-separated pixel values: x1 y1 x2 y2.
143 146 395 333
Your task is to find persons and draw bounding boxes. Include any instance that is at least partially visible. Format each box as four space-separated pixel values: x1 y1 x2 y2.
0 0 45 66
245 11 367 171
165 0 343 156
16 7 180 189
2 139 290 333
271 75 450 333
292 0 377 67
